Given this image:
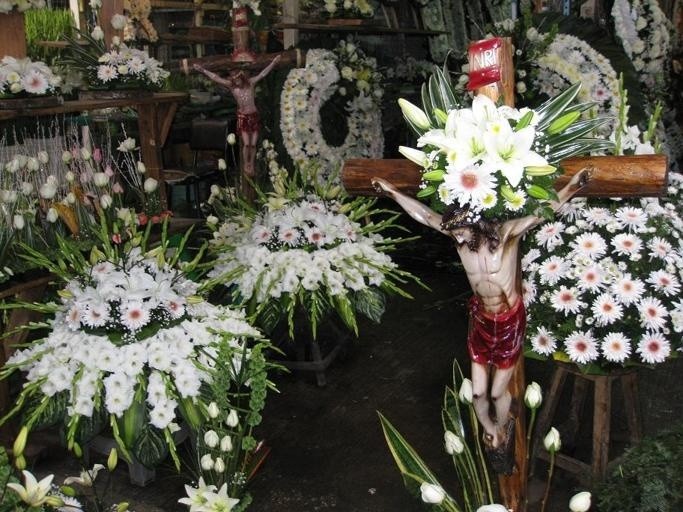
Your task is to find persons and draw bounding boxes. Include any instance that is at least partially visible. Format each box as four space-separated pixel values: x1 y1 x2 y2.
190 53 281 179
369 163 593 478
